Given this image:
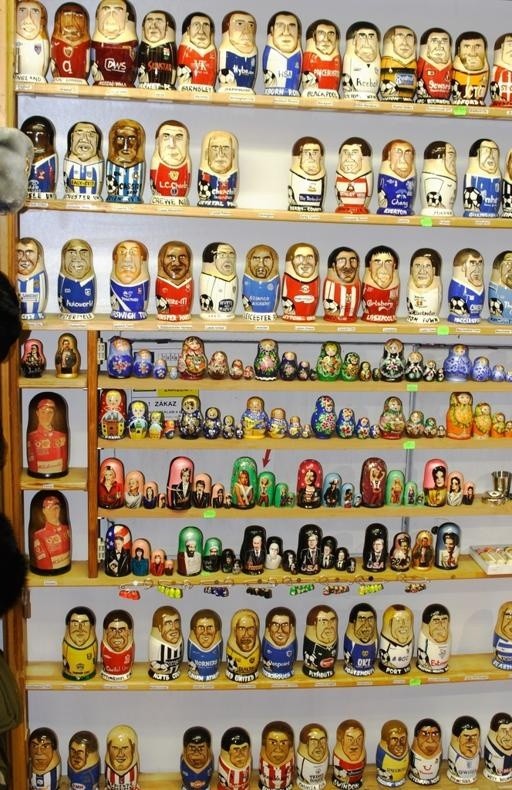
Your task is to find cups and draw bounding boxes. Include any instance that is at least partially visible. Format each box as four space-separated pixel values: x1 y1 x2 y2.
492 471 512 498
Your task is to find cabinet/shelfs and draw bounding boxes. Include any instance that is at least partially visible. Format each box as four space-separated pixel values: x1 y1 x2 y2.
0 588 512 789
2 0 511 333
99 330 512 585
5 336 96 581
